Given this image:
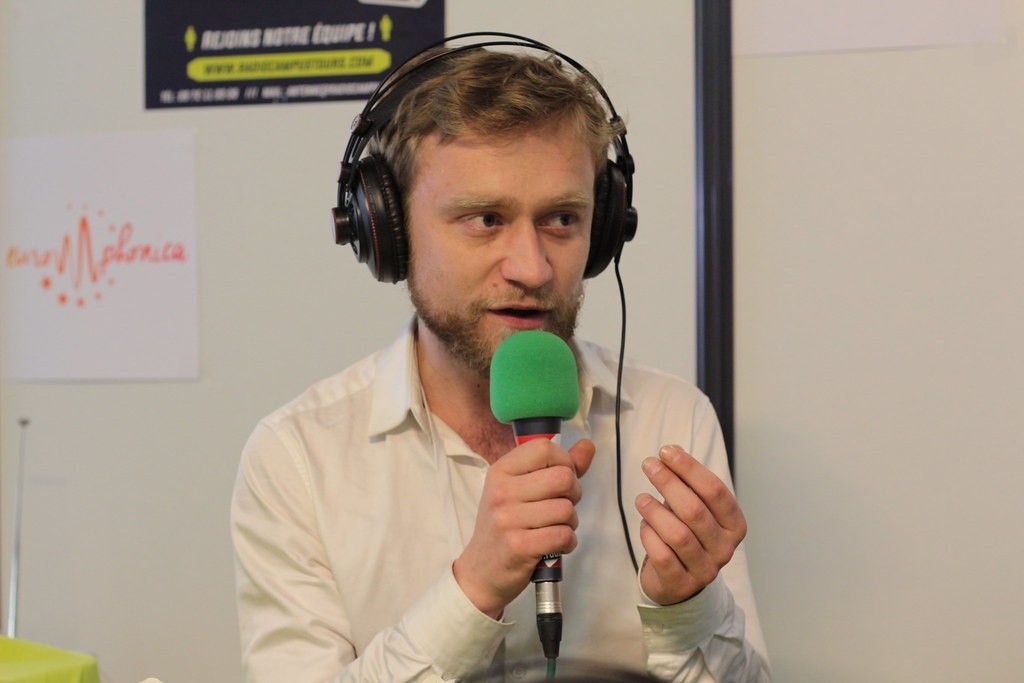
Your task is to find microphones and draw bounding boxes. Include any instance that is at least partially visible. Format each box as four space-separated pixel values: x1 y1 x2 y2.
489 329 581 658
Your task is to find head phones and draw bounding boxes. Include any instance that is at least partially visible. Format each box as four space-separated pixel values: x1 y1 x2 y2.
332 32 639 290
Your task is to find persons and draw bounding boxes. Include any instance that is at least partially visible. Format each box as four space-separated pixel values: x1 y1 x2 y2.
227 46 769 683
